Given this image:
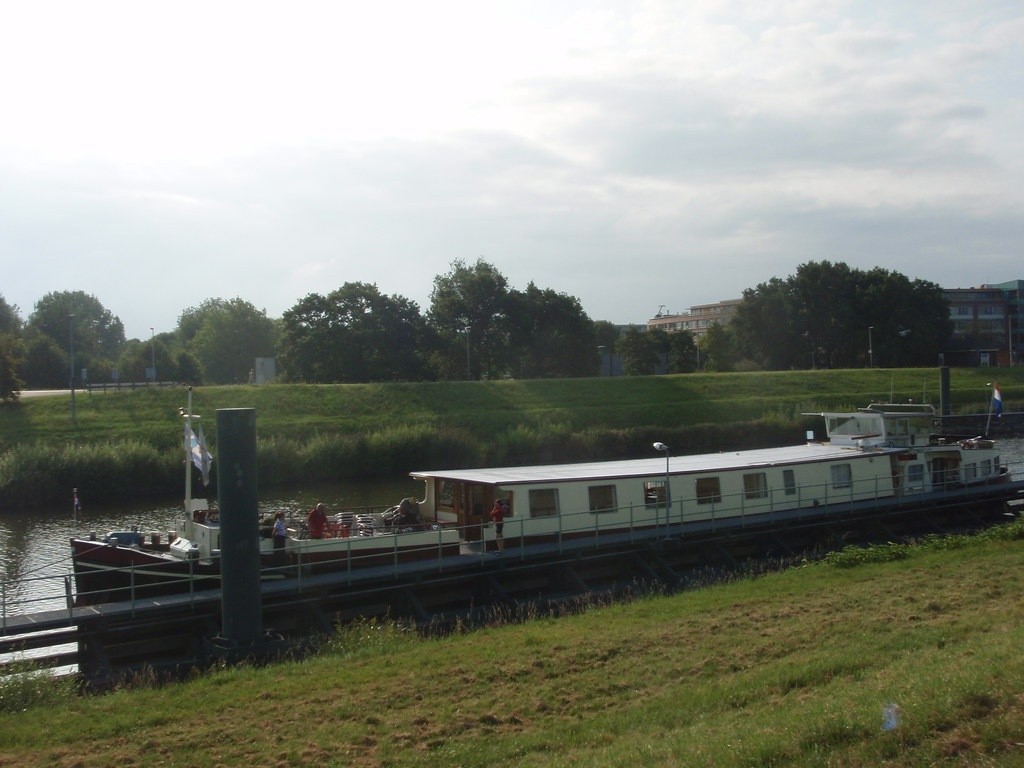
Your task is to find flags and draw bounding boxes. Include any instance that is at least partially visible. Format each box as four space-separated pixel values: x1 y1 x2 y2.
183 422 213 472
991 381 1004 418
198 423 210 487
74 495 82 511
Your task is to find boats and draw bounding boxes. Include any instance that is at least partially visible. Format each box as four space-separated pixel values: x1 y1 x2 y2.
68 348 1014 664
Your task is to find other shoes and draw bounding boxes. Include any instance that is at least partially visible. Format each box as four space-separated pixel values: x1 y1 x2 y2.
495 552 503 555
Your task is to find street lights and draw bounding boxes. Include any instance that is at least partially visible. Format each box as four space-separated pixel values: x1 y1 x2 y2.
868 325 876 369
651 441 670 539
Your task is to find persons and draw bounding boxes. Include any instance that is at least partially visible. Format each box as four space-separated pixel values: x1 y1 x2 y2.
490 499 506 556
396 497 426 532
271 512 288 548
307 503 333 540
